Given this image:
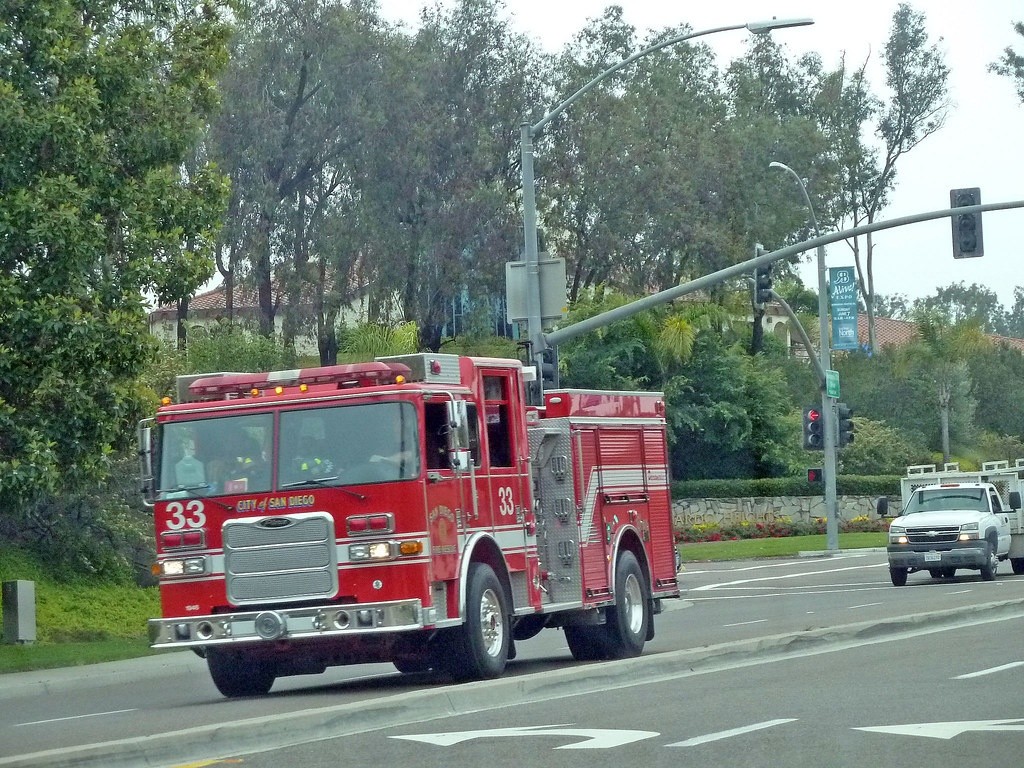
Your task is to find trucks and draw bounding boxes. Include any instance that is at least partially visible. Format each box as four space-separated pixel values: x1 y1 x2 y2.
876 457 1024 585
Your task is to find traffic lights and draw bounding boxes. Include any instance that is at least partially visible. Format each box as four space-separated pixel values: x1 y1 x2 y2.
754 242 773 310
808 468 823 482
521 17 816 367
803 405 824 450
835 403 855 448
536 344 558 390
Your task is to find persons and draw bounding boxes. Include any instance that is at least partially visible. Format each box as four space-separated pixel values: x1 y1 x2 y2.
369 417 445 471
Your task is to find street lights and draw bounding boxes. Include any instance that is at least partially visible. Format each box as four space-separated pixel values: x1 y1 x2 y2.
766 160 840 549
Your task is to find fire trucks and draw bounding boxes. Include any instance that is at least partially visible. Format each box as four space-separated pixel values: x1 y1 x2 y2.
136 352 682 699
